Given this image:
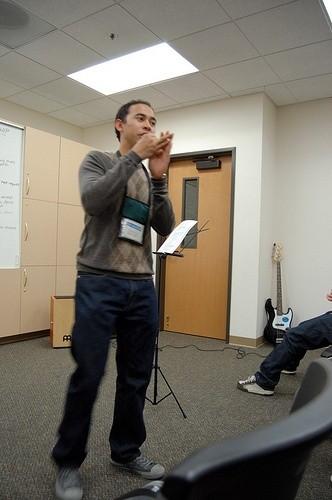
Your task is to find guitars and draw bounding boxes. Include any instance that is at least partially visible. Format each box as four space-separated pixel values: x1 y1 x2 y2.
262 242 294 345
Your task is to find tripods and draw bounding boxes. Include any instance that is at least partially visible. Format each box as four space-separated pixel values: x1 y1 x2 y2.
147 221 212 421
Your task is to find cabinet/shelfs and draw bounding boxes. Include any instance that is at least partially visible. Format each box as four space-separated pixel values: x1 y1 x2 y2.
0 125 100 344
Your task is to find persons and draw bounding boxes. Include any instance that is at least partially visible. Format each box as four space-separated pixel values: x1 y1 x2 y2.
50 97 174 500
237 287 332 395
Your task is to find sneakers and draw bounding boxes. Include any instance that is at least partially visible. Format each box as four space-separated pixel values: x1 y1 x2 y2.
281 368 298 374
54 466 83 500
237 375 275 396
111 454 166 479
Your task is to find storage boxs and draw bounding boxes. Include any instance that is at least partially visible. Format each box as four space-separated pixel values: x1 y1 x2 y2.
49 296 75 348
